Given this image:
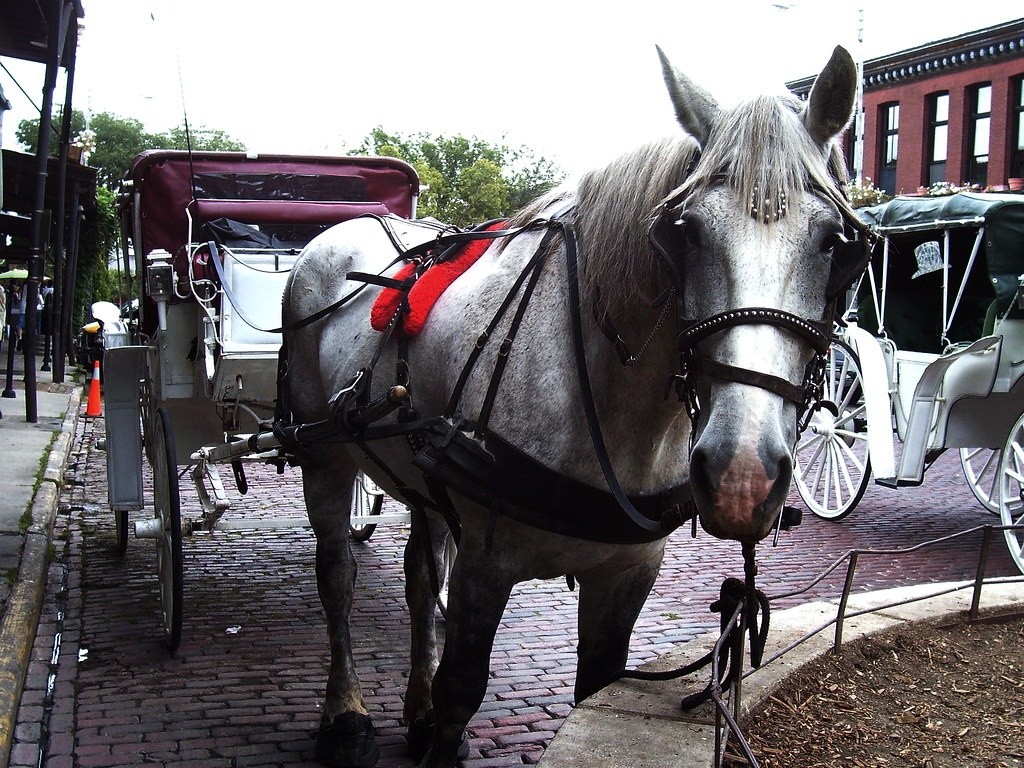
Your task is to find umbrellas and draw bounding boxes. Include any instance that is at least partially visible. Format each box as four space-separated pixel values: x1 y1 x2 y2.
0 269 28 278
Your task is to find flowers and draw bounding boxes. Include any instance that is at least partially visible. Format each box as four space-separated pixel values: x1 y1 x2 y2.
918 180 995 196
846 175 893 210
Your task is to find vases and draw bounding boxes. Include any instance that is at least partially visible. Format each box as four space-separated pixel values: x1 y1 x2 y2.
1008 178 1024 191
916 188 928 194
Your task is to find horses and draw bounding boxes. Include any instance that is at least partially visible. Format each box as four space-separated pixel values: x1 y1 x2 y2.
279 46 867 767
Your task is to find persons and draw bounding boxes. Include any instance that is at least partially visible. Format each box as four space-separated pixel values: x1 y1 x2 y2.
0 280 53 340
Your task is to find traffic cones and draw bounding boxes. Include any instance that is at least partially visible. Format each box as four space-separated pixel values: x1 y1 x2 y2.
78 360 104 419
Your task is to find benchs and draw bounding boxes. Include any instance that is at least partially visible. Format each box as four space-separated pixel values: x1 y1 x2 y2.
857 270 1023 440
131 184 410 406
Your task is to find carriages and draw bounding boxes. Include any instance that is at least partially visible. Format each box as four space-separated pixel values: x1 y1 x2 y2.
99 45 855 768
